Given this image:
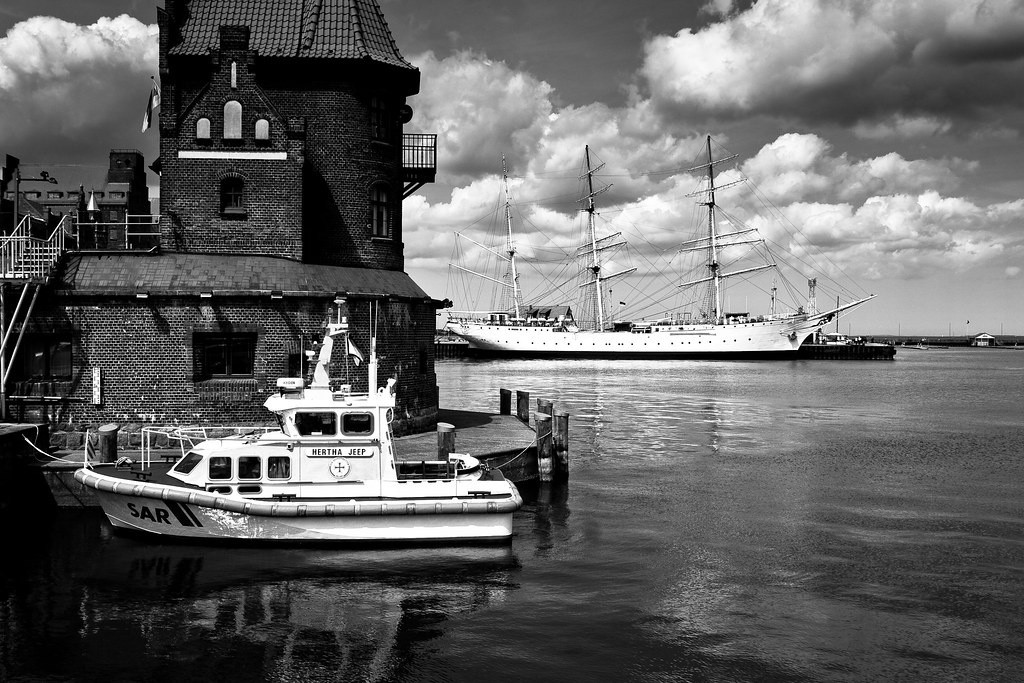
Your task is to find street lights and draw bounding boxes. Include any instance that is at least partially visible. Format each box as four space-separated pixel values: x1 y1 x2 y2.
13 167 58 230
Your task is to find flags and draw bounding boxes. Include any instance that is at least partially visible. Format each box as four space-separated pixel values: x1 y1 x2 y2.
142 81 161 134
344 336 365 366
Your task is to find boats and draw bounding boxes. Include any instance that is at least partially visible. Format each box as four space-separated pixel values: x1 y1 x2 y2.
73 289 522 552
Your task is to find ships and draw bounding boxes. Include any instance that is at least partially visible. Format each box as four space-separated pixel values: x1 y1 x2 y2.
447 133 878 358
